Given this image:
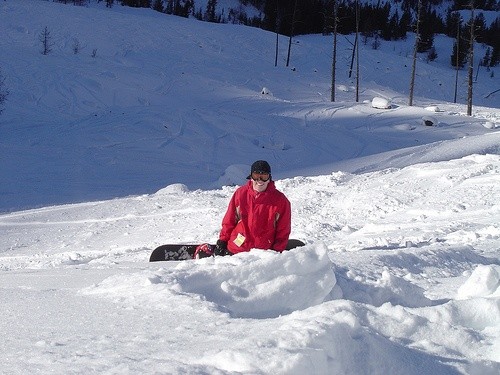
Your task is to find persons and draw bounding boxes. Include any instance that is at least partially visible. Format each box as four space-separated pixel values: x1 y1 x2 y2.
195 160 291 259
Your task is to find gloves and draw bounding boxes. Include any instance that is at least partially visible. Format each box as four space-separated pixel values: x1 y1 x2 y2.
214 239 228 256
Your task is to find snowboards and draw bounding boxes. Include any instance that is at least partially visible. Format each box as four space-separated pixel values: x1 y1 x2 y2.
150 239 305 262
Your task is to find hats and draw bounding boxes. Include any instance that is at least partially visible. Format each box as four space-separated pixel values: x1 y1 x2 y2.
246 160 272 182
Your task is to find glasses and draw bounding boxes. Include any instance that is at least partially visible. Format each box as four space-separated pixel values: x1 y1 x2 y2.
251 172 271 182
